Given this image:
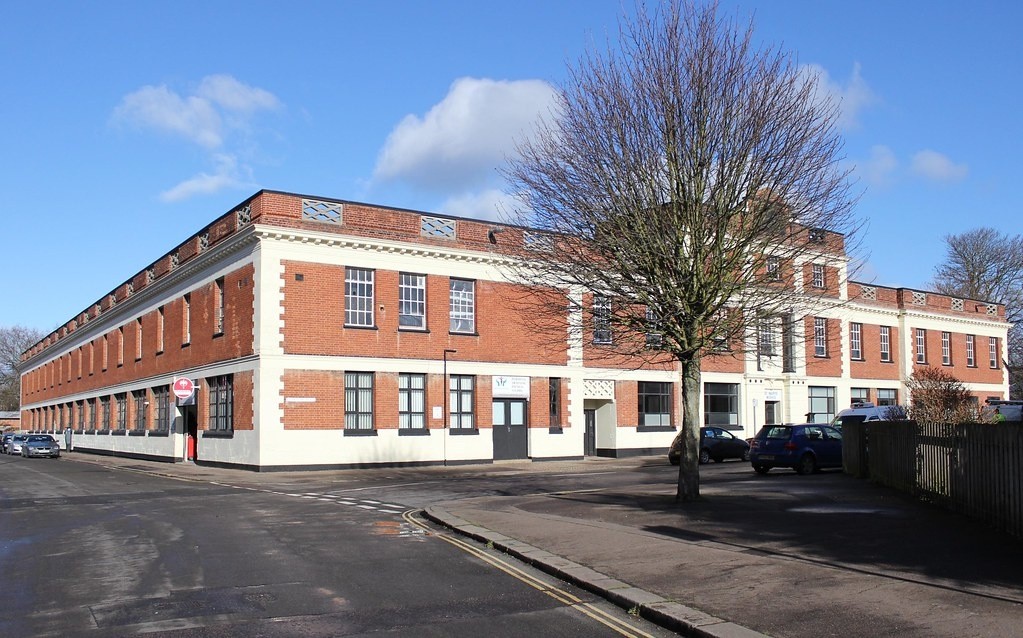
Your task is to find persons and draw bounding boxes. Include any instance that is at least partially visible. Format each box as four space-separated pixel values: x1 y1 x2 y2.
991 409 1005 424
64 427 71 454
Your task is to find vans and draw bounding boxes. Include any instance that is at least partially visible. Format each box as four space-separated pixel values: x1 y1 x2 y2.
829 401 908 435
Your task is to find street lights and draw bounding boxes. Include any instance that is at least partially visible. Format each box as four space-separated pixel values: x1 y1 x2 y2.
443 349 457 467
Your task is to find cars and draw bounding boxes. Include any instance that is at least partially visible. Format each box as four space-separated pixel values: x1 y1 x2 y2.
20 435 60 458
668 425 751 466
0 432 30 455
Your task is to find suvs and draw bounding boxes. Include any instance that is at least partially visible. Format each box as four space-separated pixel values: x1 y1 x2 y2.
747 422 870 476
